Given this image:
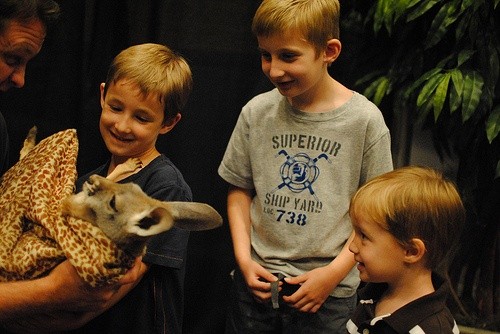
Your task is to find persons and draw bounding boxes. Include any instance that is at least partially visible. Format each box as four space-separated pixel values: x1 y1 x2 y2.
217 0 395 332
0 0 146 331
68 43 194 334
346 166 462 333
